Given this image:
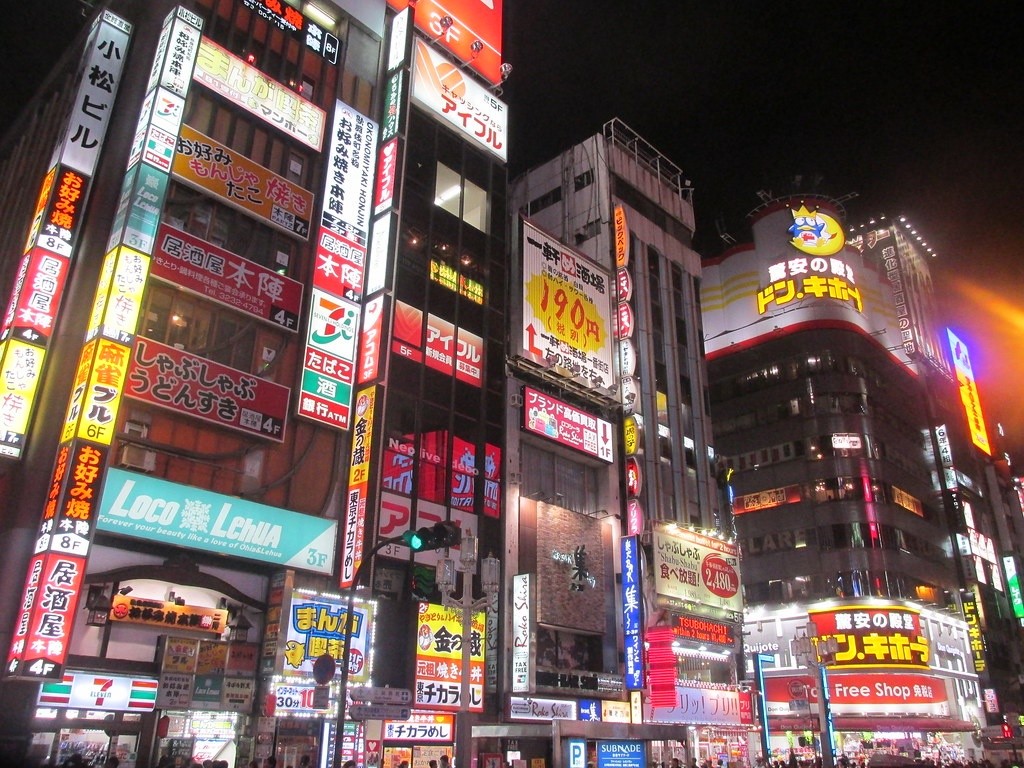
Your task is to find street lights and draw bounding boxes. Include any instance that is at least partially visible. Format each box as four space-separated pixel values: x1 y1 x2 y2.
436 536 501 767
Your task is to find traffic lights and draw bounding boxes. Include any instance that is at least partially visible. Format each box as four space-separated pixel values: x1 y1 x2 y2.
402 522 462 552
1001 722 1013 740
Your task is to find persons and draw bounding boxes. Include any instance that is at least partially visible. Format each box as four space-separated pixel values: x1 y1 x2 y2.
2 724 1024 768
